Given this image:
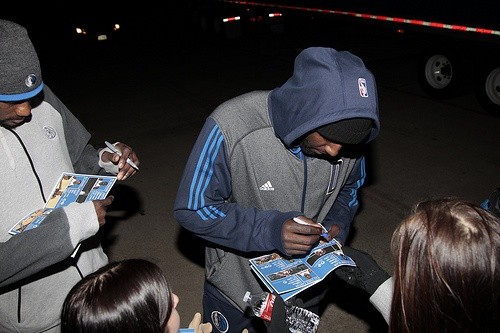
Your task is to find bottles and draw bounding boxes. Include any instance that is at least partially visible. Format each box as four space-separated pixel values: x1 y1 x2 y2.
242 292 320 333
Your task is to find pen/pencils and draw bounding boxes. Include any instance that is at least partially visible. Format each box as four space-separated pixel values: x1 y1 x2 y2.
293 217 309 227
105 140 140 172
179 327 194 332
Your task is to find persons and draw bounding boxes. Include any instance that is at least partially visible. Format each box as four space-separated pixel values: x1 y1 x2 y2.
61 259 248 333
389 198 500 333
173 46 388 333
0 20 140 333
